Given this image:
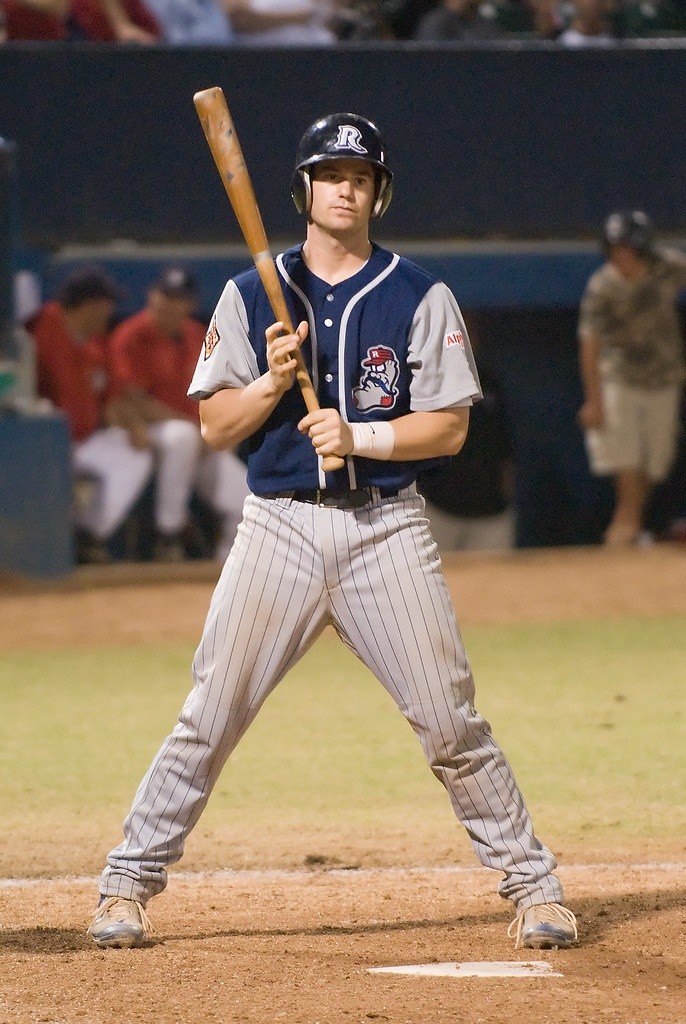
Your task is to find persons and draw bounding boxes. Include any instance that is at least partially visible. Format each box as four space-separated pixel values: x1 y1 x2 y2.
32 265 249 565
579 210 685 550
88 114 579 950
0 1 620 42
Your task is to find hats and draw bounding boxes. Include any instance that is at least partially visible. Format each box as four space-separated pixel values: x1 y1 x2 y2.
59 265 130 309
151 266 198 298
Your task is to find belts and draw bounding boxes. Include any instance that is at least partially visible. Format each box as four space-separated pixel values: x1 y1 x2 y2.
292 488 398 510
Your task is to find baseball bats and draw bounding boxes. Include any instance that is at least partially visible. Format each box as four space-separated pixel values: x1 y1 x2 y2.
194 86 346 472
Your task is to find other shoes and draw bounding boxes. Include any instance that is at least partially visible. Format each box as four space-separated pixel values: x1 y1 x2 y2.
68 524 116 564
152 525 195 563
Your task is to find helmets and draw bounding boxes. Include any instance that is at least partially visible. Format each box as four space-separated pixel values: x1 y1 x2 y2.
288 112 395 222
601 209 654 252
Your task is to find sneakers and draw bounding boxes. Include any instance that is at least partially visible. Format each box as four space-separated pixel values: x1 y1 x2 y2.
84 894 156 948
507 902 577 948
